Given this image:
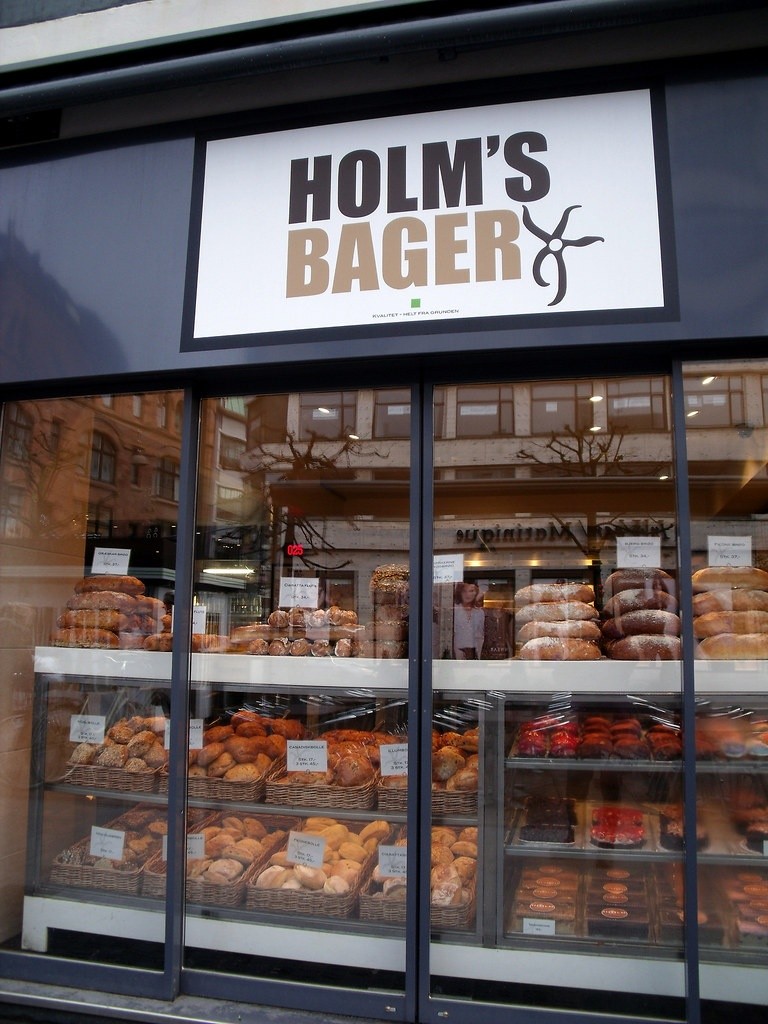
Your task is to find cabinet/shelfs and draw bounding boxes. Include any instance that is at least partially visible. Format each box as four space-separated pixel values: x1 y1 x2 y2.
20 645 768 1005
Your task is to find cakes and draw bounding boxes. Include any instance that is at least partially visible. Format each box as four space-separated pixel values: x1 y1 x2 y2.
516 790 768 854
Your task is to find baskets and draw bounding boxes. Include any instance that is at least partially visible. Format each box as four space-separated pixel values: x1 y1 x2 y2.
50 801 476 930
65 735 478 818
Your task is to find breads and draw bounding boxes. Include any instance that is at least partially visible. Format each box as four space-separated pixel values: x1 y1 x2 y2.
43 564 477 905
513 566 768 660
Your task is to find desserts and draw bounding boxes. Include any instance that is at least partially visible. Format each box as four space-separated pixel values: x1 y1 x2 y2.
512 714 768 761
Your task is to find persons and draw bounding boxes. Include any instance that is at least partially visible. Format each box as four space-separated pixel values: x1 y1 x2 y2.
484 583 514 661
453 578 485 661
641 575 675 690
